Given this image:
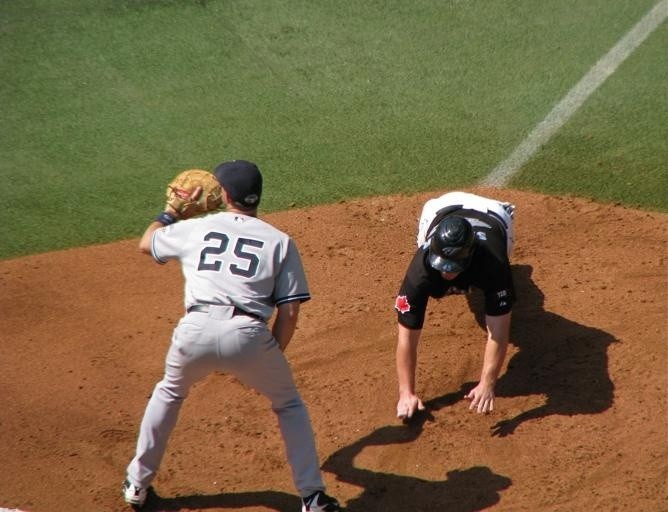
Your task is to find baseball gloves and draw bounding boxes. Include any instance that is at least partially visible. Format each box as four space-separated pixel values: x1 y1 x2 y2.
165 170 223 218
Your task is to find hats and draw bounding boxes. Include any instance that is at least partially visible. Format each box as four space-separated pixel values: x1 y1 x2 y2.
213 160 263 206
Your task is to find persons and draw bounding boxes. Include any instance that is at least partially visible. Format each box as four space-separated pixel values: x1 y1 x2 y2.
392 189 516 420
120 159 341 512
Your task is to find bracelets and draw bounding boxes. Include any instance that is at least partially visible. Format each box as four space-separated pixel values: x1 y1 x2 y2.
153 210 176 227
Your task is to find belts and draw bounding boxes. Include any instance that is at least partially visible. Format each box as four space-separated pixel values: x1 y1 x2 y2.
187 305 256 317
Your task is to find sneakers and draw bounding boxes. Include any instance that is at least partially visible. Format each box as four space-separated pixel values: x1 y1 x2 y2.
301 489 339 512
122 479 147 505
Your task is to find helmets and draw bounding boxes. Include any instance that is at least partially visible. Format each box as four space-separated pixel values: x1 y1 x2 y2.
429 215 473 272
